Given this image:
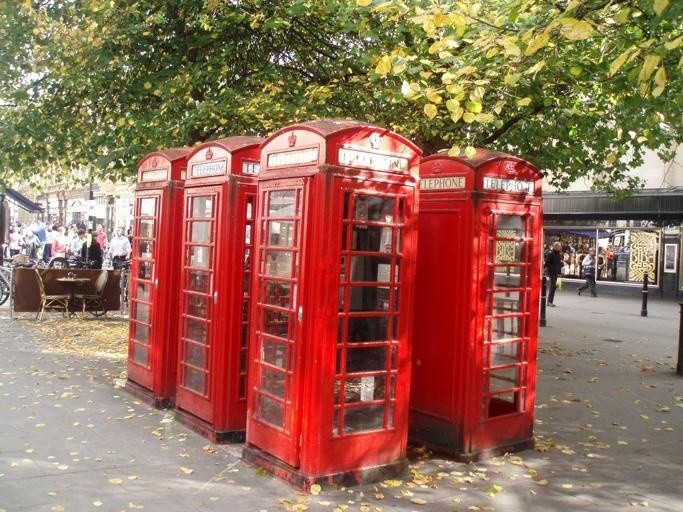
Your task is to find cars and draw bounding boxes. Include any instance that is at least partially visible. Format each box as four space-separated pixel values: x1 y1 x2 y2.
609 245 629 261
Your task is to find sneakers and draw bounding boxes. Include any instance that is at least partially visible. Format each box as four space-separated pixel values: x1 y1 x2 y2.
577 288 599 297
546 301 556 307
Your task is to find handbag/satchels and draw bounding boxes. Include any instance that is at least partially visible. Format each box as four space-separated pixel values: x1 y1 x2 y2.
556 278 563 291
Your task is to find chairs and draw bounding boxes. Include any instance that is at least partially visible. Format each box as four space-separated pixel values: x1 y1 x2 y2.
32 269 111 324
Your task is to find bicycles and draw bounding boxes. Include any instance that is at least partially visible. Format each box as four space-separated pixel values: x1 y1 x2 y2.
0 257 132 317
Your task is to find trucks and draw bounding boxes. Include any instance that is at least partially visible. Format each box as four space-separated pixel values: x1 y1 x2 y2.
611 230 631 247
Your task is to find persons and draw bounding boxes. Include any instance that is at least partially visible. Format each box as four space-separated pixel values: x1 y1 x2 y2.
9 218 134 270
544 241 561 307
605 246 615 277
576 247 598 297
